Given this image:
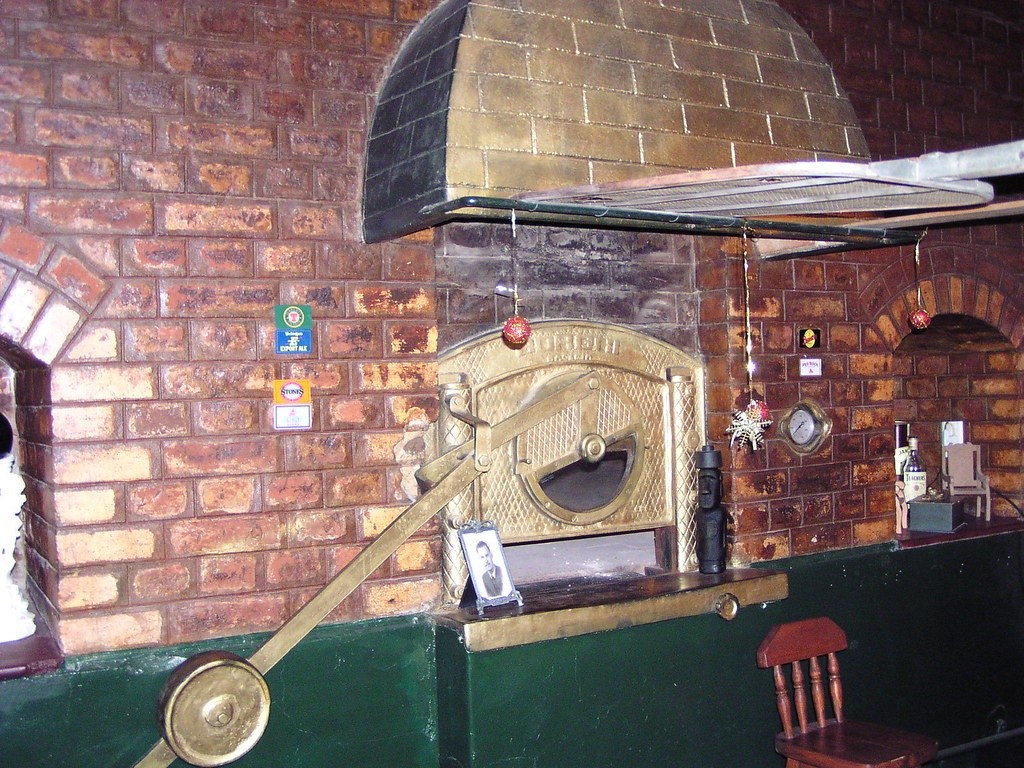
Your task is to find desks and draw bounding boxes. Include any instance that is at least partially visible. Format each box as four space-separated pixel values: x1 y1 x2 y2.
906 494 967 534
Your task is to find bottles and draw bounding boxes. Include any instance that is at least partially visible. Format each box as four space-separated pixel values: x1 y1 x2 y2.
903 437 927 519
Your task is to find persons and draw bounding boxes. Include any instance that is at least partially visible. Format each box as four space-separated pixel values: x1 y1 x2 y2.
695 469 734 576
475 541 503 597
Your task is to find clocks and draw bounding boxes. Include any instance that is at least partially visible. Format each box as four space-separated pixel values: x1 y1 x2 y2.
777 399 832 454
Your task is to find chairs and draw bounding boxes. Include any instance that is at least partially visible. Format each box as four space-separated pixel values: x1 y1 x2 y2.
941 442 991 521
757 617 938 768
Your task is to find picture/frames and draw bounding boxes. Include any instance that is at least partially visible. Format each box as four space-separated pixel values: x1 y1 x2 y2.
456 519 524 615
